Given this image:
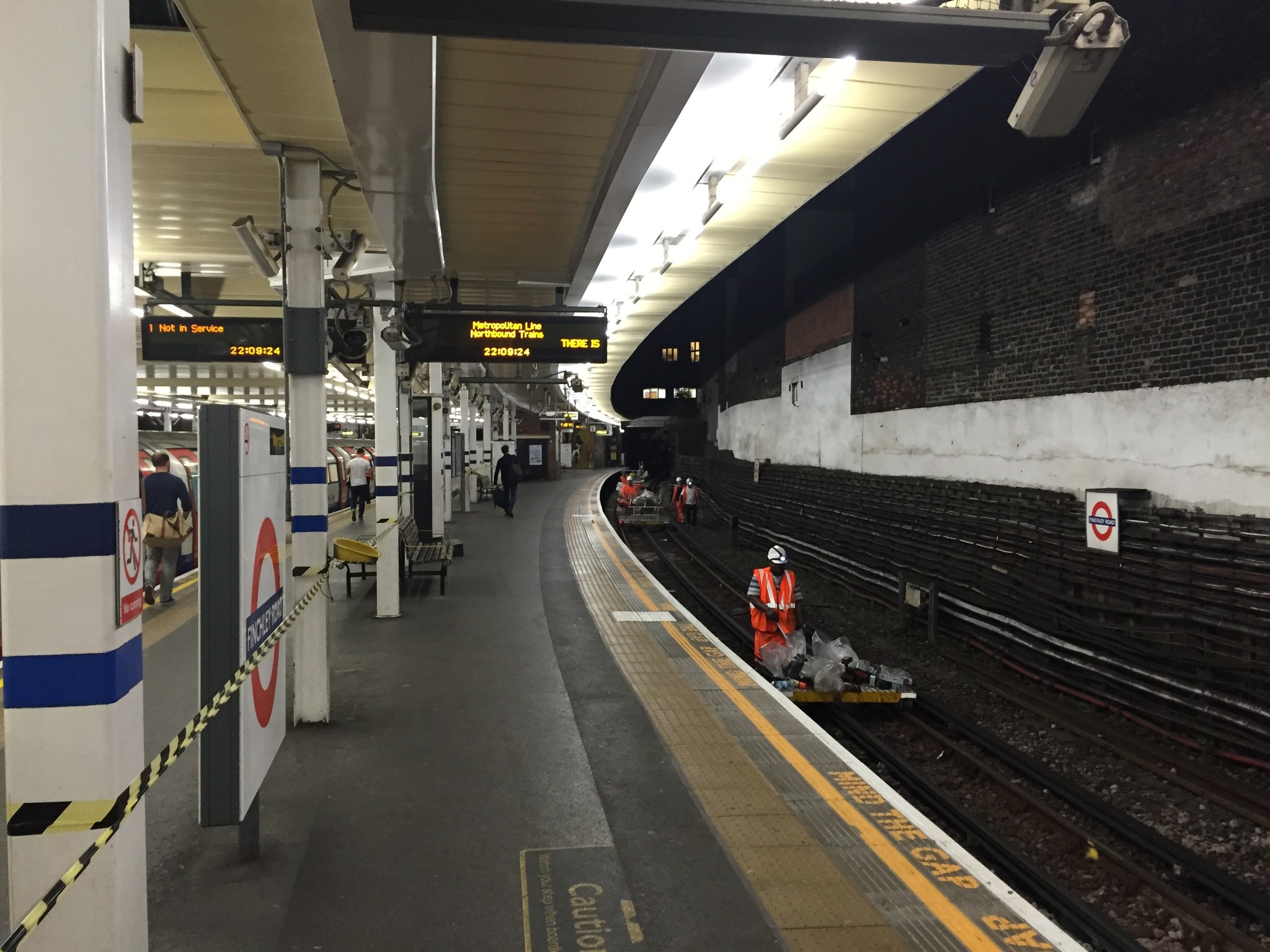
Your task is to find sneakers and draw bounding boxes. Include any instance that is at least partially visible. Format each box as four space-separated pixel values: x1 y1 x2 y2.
160 596 175 606
358 517 364 522
505 509 514 518
144 583 155 606
352 510 356 521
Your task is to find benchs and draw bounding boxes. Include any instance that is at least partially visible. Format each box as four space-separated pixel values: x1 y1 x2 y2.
343 533 376 597
479 475 504 500
400 513 452 596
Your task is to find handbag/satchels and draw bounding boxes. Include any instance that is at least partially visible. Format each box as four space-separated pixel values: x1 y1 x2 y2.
511 462 524 482
492 488 508 509
141 508 194 549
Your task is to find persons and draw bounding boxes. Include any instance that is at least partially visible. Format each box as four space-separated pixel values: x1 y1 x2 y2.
668 478 687 526
637 465 649 480
619 470 630 486
572 446 580 467
576 435 582 450
750 545 808 671
620 476 641 505
137 451 191 605
355 450 374 504
679 478 701 530
345 448 372 522
493 445 524 518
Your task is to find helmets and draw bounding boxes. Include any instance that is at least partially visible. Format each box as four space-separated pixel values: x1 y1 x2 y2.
768 544 788 565
676 477 682 482
620 469 626 473
627 476 633 481
685 478 693 484
